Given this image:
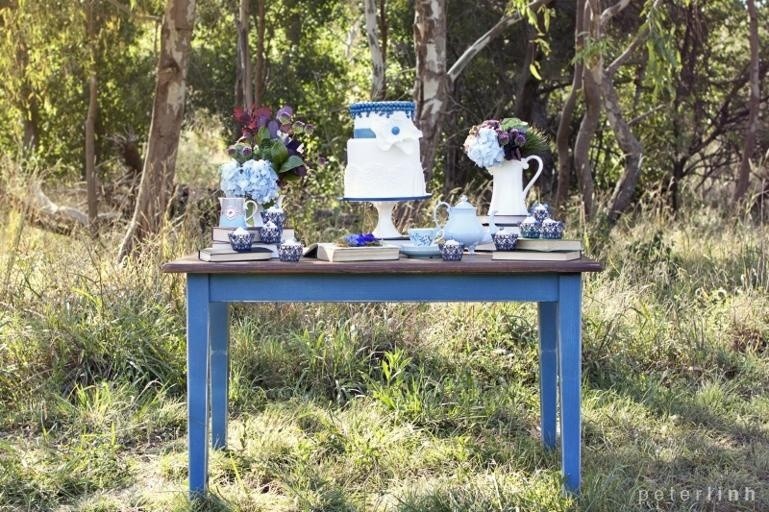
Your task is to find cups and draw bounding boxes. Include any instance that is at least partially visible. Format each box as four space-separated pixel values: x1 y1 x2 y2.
407 227 445 249
217 198 258 228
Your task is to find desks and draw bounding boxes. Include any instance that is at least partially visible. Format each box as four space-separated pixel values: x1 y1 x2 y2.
157 246 606 501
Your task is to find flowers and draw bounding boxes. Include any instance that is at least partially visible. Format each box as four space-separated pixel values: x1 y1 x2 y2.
218 103 320 210
463 117 556 177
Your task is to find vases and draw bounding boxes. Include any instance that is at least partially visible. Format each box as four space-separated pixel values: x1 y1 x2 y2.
487 153 544 223
252 193 285 226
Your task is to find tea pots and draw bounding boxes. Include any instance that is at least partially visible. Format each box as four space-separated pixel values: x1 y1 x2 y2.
433 194 499 249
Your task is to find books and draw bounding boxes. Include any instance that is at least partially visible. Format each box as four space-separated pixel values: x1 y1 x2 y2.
199 227 280 262
492 238 582 261
316 243 401 262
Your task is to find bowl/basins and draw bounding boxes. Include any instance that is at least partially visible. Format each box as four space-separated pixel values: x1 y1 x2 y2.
540 222 565 239
438 242 465 261
260 207 288 228
490 233 518 252
228 232 256 251
529 208 551 227
518 221 542 239
277 243 304 262
258 226 282 245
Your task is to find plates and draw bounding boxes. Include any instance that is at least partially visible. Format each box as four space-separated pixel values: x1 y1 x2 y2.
399 248 442 259
